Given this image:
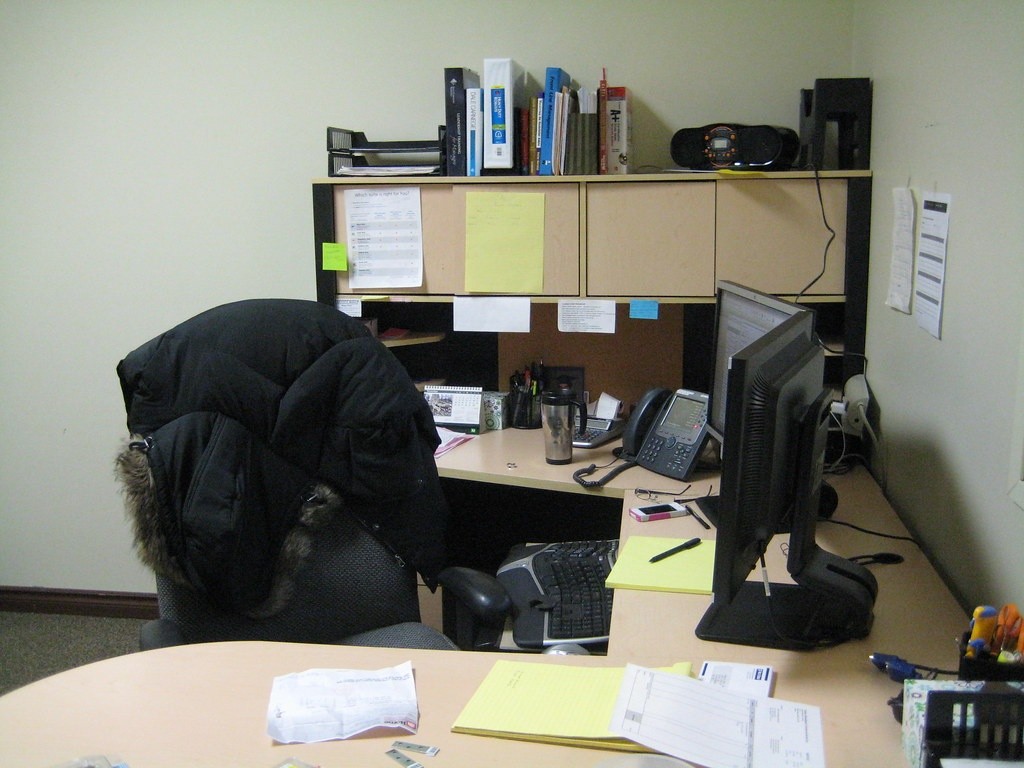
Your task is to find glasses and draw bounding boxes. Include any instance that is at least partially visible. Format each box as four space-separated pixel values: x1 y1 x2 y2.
635 484 712 505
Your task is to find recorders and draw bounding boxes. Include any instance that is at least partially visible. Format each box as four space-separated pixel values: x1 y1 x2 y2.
670 123 799 171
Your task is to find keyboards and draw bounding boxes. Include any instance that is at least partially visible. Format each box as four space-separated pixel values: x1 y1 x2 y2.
497 539 619 650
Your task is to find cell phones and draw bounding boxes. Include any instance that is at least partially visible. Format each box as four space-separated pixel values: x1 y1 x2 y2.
629 502 686 522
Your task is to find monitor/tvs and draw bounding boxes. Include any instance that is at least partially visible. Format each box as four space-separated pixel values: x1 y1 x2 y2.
695 280 879 653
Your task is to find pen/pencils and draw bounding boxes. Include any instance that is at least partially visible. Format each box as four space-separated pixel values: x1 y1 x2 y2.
685 503 711 530
512 355 544 428
648 537 701 564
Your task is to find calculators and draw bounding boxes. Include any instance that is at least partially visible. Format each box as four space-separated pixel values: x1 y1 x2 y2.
571 414 625 449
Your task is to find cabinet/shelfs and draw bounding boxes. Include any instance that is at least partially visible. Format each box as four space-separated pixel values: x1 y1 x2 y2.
310 168 874 304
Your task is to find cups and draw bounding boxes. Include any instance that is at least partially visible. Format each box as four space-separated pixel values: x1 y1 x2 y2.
958 631 1024 682
509 375 543 429
541 391 587 465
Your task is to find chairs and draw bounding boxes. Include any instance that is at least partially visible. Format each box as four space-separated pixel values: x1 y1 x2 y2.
116 296 514 651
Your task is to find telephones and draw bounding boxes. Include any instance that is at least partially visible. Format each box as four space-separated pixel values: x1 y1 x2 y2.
621 382 711 482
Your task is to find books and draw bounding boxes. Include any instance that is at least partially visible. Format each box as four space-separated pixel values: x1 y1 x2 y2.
444 57 631 175
604 534 715 594
451 658 692 754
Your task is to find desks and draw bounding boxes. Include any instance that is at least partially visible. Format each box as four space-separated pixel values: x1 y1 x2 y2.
0 639 1024 768
433 427 886 499
606 488 974 655
